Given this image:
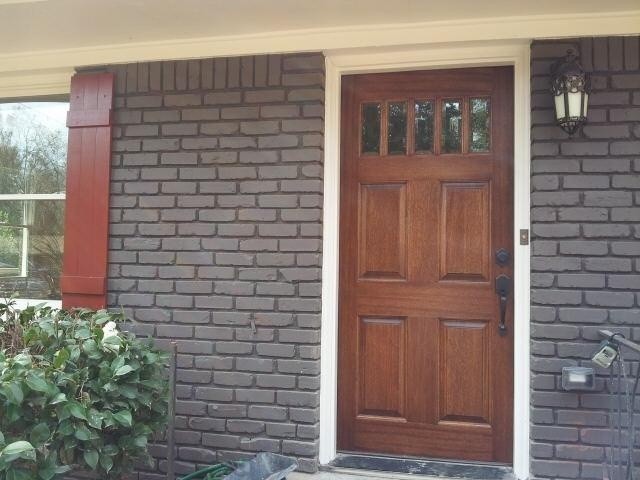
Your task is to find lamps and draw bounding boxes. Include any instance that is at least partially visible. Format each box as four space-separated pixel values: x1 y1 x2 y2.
550 45 591 137
